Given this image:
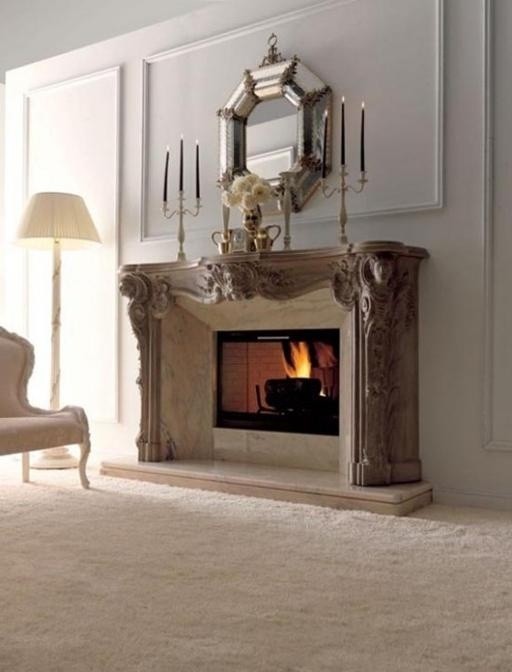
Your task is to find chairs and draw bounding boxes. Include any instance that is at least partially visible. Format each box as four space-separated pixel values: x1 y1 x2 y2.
0 324 92 489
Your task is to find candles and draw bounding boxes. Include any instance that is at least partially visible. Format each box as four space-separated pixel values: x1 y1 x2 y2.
162 136 201 203
320 98 369 180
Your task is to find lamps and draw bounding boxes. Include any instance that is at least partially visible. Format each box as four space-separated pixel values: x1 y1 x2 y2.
15 189 102 471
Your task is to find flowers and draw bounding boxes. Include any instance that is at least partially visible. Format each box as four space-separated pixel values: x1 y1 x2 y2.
223 175 274 211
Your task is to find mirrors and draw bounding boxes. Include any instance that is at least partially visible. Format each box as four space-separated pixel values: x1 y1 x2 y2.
214 30 335 214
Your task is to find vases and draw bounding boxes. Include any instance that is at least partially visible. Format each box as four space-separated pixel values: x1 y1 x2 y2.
242 208 261 254
